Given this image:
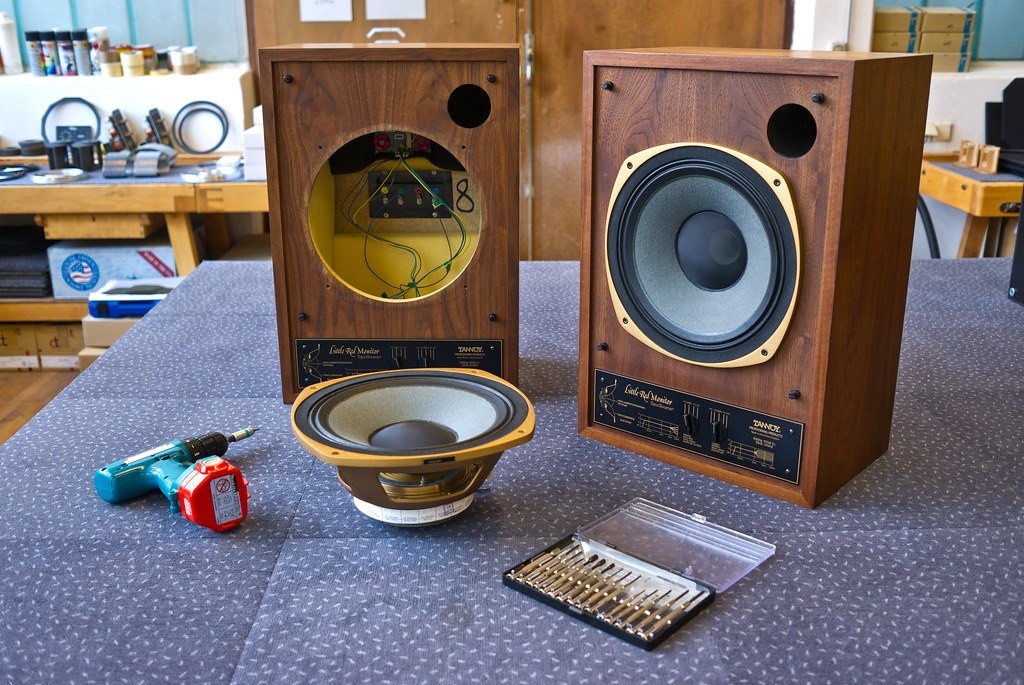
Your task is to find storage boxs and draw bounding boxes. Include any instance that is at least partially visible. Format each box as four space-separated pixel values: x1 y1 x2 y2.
916 6 977 33
873 6 925 32
918 31 974 53
82 313 140 348
34 323 86 372
921 52 971 72
872 31 920 52
78 347 108 374
0 325 41 373
46 223 210 298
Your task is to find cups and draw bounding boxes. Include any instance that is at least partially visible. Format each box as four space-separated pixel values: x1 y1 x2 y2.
958 140 978 168
976 145 1000 174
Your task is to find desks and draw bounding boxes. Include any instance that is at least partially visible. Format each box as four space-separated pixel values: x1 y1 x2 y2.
0 152 269 276
0 258 1024 685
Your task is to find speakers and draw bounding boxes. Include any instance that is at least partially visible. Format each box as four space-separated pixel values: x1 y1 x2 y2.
259 43 520 395
577 44 934 512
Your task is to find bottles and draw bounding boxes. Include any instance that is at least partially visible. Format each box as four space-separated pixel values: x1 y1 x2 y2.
0 12 199 78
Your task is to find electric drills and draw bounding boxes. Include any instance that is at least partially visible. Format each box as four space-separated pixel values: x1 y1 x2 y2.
94 427 261 534
